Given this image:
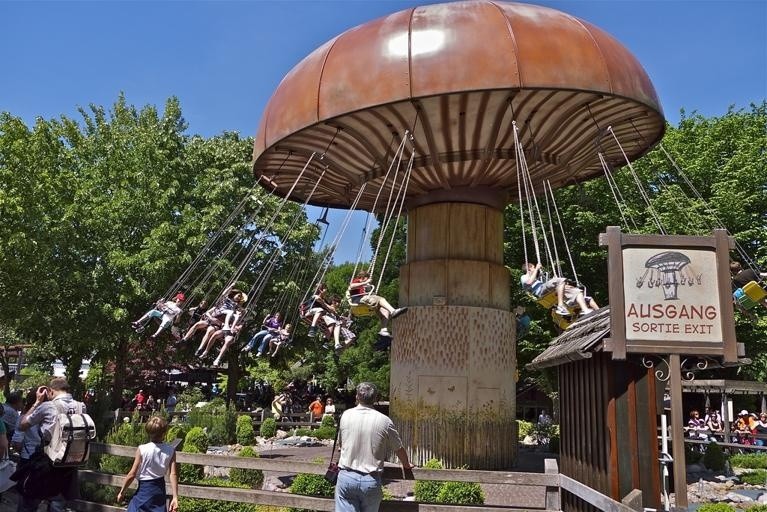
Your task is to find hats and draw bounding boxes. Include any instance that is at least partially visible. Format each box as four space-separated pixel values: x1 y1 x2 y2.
738 410 758 420
176 293 186 301
358 272 369 278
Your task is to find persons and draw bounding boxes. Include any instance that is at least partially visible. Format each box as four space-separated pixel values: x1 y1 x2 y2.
334 381 416 512
347 270 408 339
515 263 601 337
538 410 551 425
730 261 767 322
686 407 767 453
117 415 178 512
131 283 356 429
0 364 87 512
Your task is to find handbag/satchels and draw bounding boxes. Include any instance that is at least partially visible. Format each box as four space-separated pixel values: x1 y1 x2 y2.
324 462 339 486
0 460 17 494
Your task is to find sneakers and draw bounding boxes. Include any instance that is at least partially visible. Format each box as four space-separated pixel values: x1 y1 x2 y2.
308 330 354 352
556 306 571 315
129 321 222 368
244 344 278 357
391 307 408 319
579 308 594 315
376 330 394 338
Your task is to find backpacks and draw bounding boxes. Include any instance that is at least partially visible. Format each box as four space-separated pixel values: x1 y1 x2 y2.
41 399 97 473
